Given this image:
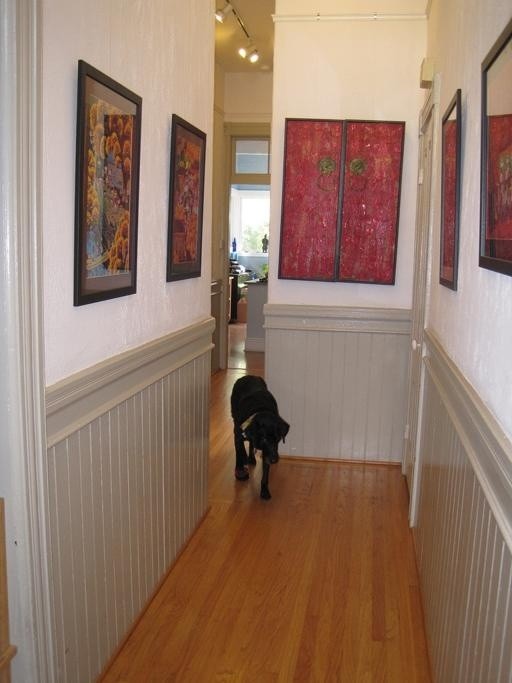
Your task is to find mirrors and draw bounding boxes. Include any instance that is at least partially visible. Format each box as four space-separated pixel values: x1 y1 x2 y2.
479 18 512 278
438 88 462 292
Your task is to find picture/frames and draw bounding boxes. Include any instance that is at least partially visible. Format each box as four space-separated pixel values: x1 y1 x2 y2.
166 114 206 282
73 59 142 307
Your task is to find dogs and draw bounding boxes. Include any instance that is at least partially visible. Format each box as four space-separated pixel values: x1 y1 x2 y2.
230 374 290 501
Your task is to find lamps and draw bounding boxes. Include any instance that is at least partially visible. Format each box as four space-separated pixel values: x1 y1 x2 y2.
215 3 259 63
